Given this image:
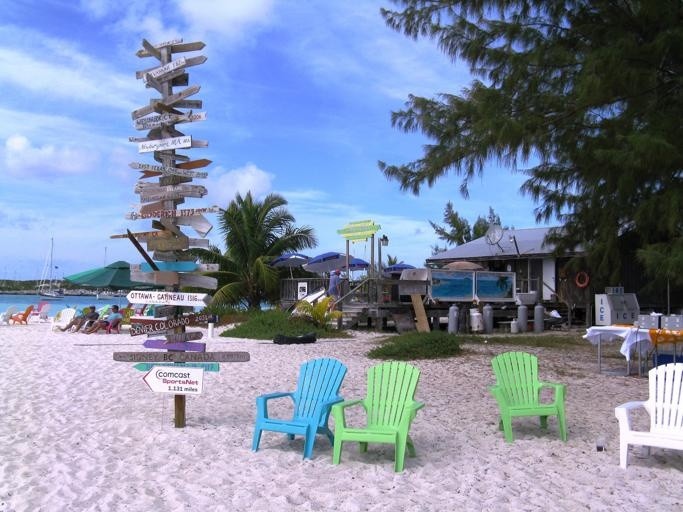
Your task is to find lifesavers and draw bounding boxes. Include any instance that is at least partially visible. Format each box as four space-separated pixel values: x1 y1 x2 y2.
576 271 590 288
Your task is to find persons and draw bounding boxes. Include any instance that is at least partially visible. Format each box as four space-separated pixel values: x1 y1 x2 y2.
78 304 122 334
57 305 98 332
327 269 340 311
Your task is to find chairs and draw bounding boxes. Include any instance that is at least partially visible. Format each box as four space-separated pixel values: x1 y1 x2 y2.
488 351 567 442
0 301 148 334
332 362 425 472
615 362 683 469
252 357 348 460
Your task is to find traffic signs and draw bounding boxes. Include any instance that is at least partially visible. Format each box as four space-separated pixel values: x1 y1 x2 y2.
112 351 251 367
108 35 221 293
142 339 205 353
142 365 205 395
129 312 213 338
163 331 203 346
132 363 219 374
125 289 209 312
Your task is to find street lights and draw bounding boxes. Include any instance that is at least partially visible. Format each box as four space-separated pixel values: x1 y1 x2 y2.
377 235 389 303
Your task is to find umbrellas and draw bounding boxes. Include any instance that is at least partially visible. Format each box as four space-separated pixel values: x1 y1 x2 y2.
442 261 484 271
381 263 416 281
62 260 164 310
339 256 369 281
268 253 308 280
301 251 353 280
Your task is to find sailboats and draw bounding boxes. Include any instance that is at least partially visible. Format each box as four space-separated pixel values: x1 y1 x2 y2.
33 236 65 298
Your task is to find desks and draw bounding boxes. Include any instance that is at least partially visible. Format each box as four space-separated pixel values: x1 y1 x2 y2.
594 323 683 376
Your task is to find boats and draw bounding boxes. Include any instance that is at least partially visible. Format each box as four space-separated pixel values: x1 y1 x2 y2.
96 293 114 300
